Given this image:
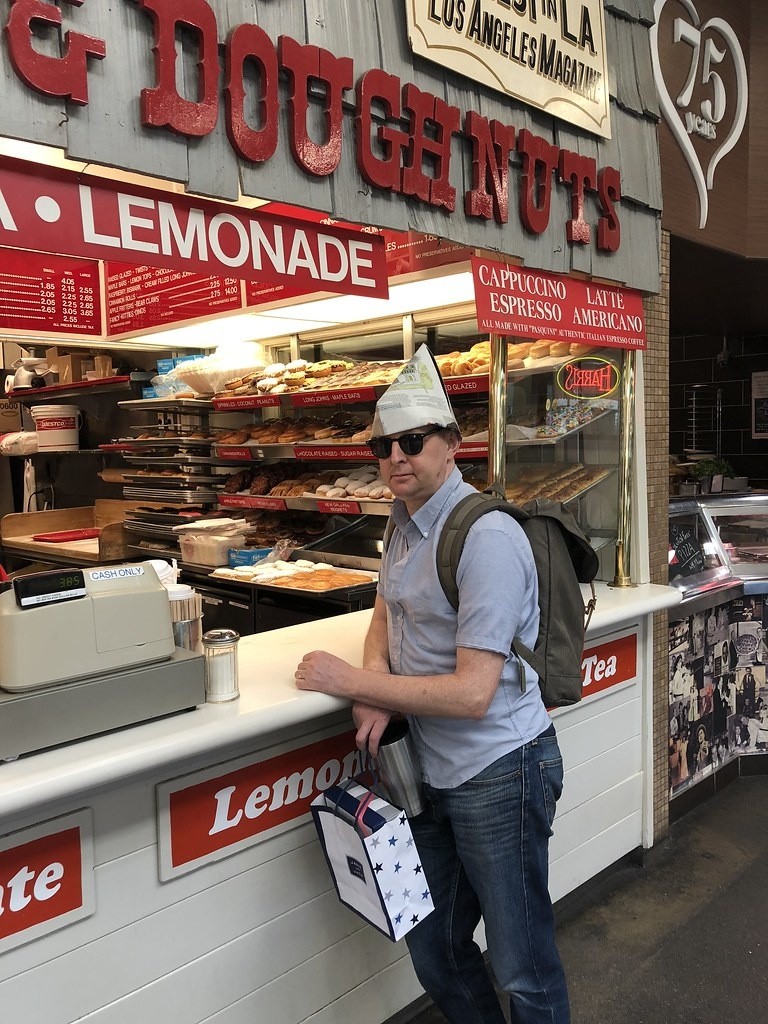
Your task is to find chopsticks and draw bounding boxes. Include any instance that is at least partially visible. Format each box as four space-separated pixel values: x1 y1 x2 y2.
169 593 202 622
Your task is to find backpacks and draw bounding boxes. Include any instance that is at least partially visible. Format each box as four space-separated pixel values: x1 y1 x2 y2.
436 482 598 707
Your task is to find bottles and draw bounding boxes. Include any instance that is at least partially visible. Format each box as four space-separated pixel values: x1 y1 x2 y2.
201 628 240 705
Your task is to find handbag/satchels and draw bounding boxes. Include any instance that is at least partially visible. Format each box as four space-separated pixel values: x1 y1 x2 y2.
311 751 436 942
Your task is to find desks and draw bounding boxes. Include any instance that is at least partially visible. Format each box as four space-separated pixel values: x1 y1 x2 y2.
0 497 204 568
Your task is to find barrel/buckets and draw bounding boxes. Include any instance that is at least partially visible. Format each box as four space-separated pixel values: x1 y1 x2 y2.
31 405 81 452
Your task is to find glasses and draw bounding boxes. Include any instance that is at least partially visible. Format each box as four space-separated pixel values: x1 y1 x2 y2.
366 427 443 459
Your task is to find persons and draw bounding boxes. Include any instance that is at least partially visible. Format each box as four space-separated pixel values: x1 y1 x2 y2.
295 341 571 1024
670 598 768 781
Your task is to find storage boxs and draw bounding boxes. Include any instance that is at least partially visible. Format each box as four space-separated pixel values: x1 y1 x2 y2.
45 346 91 370
43 372 59 386
59 355 95 382
229 545 273 569
176 378 189 385
159 372 176 384
143 387 159 398
177 386 191 392
324 780 401 837
178 540 247 567
159 385 177 397
157 359 175 371
176 355 208 368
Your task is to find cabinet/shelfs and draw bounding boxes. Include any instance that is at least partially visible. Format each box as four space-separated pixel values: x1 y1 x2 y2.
179 571 375 637
108 399 265 563
211 344 617 612
4 372 157 470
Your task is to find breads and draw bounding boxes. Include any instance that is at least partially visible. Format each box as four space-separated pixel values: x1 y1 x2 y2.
136 340 608 591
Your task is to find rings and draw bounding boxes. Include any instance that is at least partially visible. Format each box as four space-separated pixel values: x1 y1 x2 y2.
298 670 304 678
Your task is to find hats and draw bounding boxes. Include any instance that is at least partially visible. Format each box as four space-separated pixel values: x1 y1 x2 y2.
370 343 462 438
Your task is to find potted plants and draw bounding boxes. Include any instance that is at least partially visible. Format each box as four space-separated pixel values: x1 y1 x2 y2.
691 459 736 493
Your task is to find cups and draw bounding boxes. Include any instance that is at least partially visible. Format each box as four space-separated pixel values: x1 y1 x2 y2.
173 616 202 653
81 360 95 381
95 356 112 380
